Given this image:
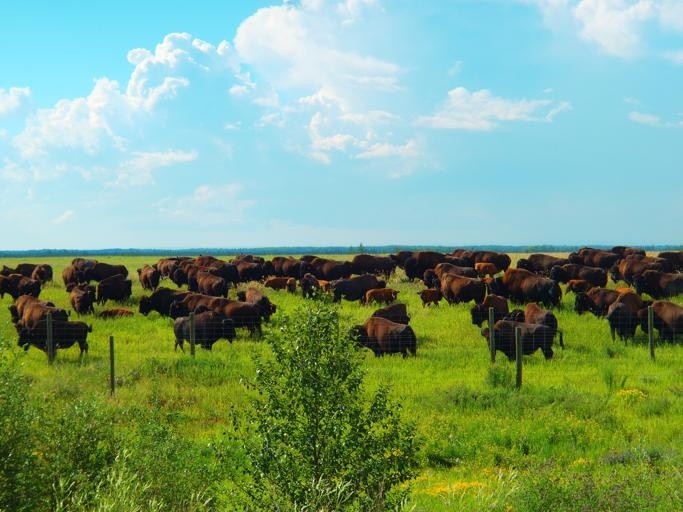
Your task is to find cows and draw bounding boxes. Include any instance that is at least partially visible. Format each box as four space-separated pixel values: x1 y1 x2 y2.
0 245 134 365
138 245 683 362
